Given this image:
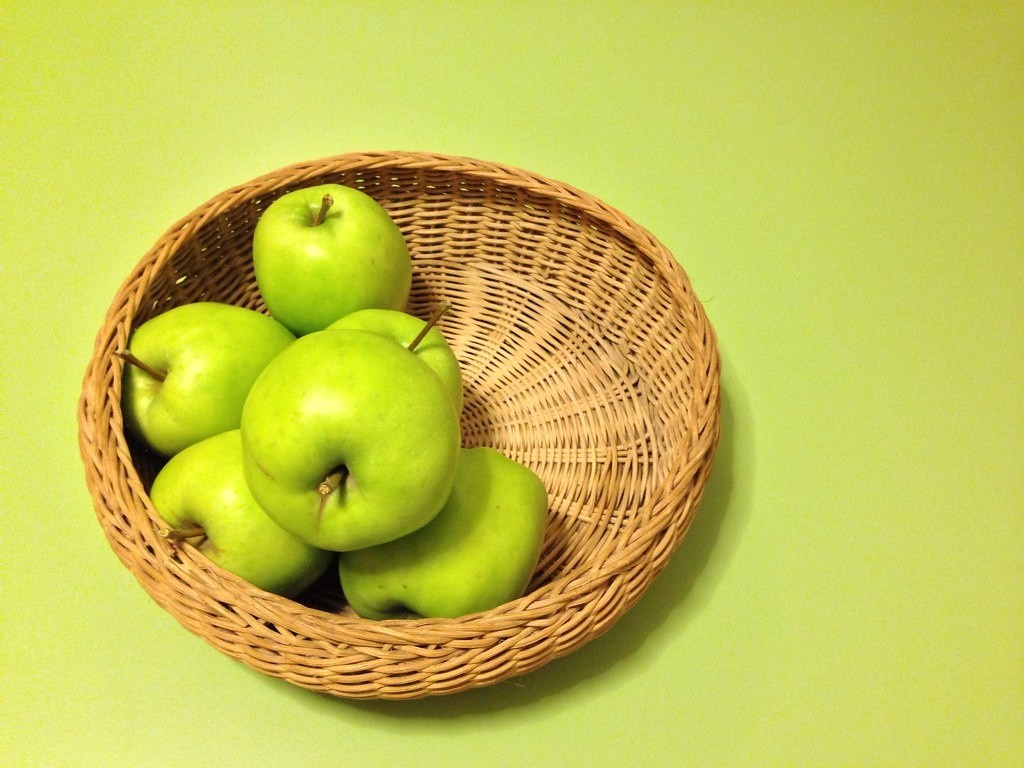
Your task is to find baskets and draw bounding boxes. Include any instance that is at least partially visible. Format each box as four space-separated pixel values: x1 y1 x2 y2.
77 150 719 701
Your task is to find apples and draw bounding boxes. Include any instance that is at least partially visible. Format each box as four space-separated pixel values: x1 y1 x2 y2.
254 183 413 338
114 300 299 460
323 300 464 424
337 447 548 621
149 427 338 602
240 329 459 552
